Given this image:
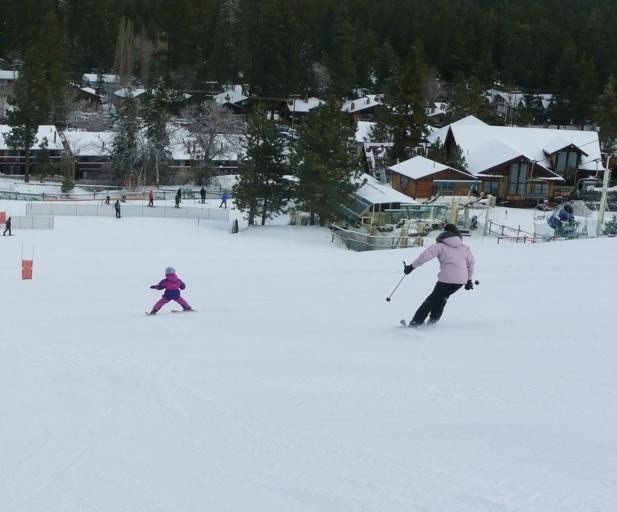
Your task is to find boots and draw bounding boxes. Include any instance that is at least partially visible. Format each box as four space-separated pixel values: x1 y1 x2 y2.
408 320 437 329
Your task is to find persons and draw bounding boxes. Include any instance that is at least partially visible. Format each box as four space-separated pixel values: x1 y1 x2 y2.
115 200 121 218
175 193 180 208
219 191 227 209
106 196 109 203
3 217 11 236
200 187 206 203
403 224 475 328
147 267 194 314
178 189 181 203
148 191 153 207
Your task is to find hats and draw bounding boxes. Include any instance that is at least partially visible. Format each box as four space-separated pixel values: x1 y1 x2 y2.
443 223 458 231
165 266 176 275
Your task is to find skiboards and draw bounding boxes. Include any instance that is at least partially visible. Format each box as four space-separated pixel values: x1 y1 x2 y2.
145 310 198 315
399 320 438 328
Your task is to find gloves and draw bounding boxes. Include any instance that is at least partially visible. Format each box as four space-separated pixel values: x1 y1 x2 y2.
150 285 156 289
465 280 473 290
403 264 414 274
